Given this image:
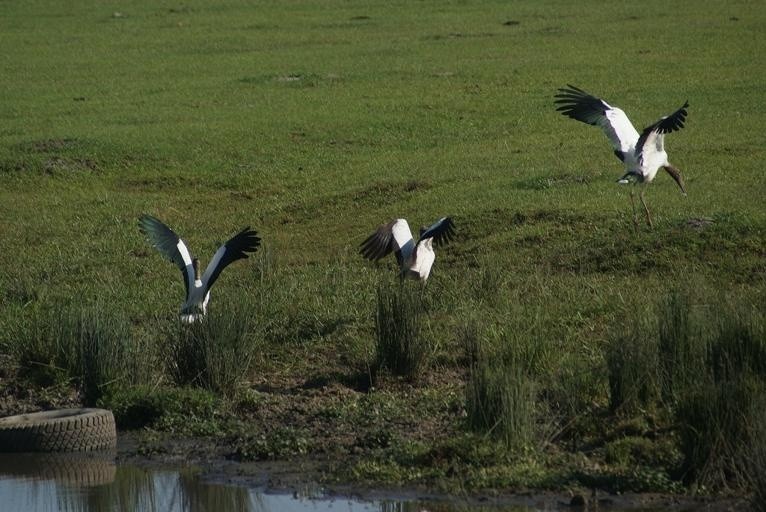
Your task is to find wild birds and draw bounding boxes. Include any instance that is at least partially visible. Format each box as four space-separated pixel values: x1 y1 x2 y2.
358 215 457 299
137 211 263 328
552 83 690 236
